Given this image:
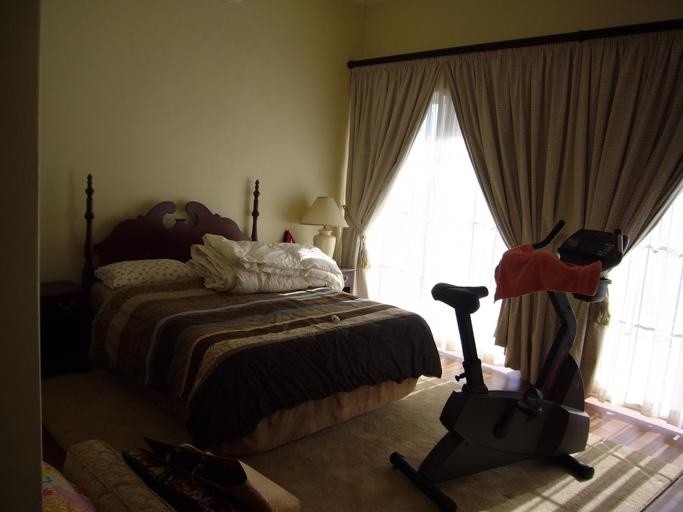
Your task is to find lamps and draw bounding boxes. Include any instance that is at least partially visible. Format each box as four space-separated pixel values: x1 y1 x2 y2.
298 197 349 260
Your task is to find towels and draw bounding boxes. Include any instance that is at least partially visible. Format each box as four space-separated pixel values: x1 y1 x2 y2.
493 243 602 301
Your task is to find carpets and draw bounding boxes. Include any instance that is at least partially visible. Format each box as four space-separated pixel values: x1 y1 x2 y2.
39 356 681 511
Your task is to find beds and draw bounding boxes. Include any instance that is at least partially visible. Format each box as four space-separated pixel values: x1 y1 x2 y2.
75 173 428 455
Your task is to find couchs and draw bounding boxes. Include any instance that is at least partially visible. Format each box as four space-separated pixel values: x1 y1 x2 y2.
40 432 303 511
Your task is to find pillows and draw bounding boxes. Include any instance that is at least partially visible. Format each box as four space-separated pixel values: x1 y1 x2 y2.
95 257 202 290
125 443 254 512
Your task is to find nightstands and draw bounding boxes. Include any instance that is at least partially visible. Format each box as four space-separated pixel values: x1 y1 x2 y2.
39 280 84 375
339 264 356 294
